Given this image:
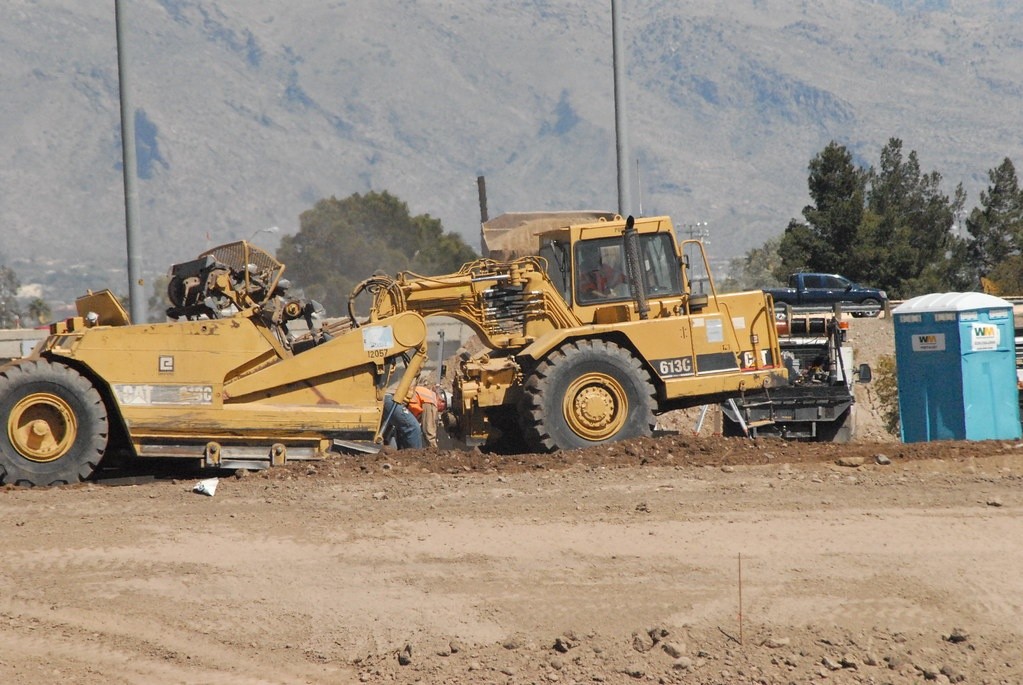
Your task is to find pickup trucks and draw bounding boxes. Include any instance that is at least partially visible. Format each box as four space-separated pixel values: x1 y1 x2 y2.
762 273 887 317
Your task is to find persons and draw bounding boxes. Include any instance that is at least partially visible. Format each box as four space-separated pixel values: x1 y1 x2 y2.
382 386 447 449
577 247 653 300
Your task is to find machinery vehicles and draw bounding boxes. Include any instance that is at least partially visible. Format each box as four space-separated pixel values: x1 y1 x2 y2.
0 0 788 488
721 302 872 442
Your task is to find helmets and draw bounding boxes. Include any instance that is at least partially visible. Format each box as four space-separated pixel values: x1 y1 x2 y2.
440 389 453 411
